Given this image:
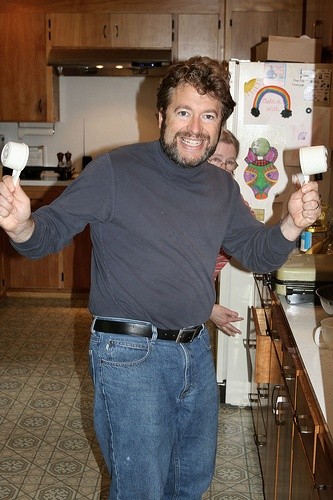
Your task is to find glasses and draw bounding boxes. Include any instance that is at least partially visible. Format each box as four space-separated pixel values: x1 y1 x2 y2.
209 158 238 171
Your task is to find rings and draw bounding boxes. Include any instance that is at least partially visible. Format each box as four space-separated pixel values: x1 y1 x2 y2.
315 200 319 209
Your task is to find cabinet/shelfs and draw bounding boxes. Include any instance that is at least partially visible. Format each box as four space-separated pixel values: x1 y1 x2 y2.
242 276 333 500
0 182 93 300
0 0 333 125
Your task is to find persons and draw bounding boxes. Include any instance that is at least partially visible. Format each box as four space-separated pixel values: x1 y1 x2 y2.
1 56 321 500
206 131 256 337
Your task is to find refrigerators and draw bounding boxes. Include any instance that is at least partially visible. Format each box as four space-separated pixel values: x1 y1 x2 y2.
215 58 333 408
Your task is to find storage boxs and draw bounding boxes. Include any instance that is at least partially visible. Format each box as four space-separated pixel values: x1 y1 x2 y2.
253 34 323 64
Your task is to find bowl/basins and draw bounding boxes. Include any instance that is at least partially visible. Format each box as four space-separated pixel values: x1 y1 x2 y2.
315 284 333 317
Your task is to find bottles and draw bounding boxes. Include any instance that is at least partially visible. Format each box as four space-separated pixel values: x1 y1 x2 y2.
57 152 72 166
300 227 312 252
305 237 333 255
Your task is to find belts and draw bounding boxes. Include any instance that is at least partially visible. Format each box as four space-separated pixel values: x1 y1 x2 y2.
91 316 204 344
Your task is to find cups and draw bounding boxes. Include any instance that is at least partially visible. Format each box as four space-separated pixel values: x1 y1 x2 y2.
58 167 72 180
314 318 333 349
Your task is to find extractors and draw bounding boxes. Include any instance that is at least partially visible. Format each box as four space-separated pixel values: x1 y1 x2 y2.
46 47 170 77
